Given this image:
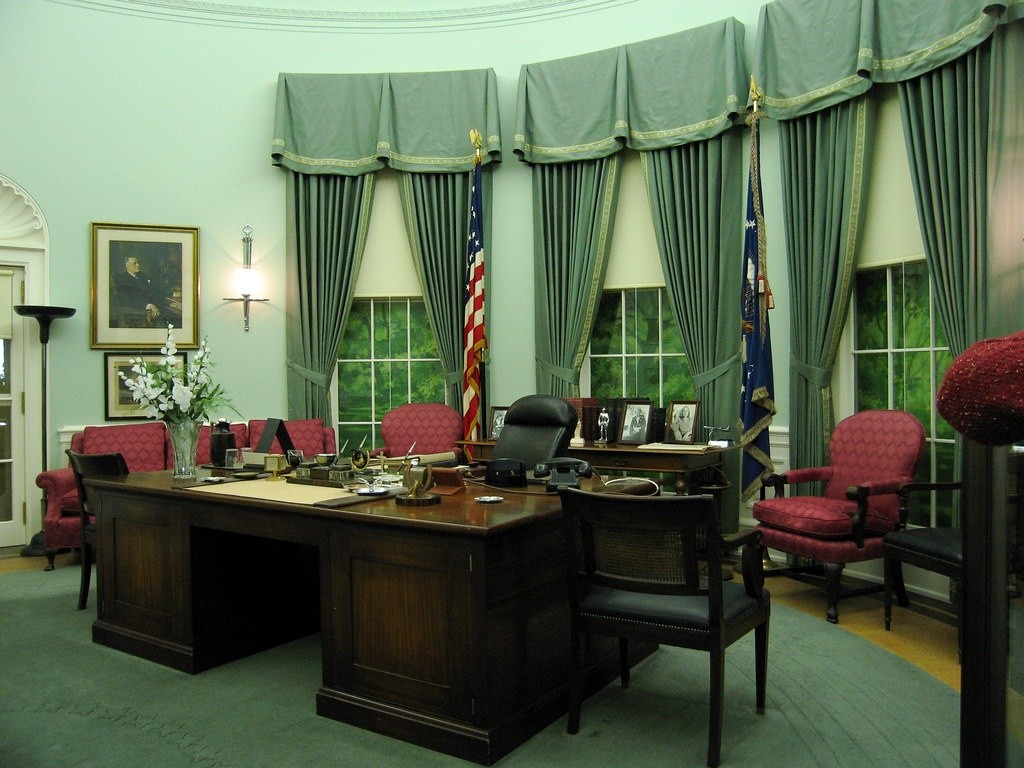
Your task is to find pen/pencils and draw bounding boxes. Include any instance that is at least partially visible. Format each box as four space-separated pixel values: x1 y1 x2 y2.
353 436 367 456
333 439 349 464
398 441 417 472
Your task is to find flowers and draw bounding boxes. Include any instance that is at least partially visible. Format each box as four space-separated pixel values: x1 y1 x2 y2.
113 318 243 444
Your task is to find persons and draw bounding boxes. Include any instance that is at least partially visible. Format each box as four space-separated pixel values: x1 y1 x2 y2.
493 414 504 430
597 408 610 442
677 405 693 440
628 407 646 441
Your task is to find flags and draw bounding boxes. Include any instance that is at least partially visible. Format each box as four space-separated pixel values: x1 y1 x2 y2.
741 110 777 506
460 153 490 464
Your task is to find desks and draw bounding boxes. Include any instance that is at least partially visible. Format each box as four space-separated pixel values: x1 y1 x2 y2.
73 465 662 768
455 439 742 534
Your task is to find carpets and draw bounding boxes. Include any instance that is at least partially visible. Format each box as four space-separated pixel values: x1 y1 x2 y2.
0 565 961 768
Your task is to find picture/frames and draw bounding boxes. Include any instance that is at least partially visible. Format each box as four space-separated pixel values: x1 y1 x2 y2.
86 220 200 352
103 351 189 421
663 401 701 446
488 406 510 441
615 400 654 445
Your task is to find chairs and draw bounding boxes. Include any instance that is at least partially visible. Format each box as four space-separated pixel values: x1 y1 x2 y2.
884 450 1024 674
165 424 248 469
369 402 463 460
35 422 166 573
492 394 578 472
557 483 771 768
751 408 927 625
250 418 337 456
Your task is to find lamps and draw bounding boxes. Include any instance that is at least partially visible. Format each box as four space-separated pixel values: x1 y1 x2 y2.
221 224 270 332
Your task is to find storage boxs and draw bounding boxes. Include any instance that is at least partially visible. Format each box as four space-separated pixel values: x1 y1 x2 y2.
310 466 330 480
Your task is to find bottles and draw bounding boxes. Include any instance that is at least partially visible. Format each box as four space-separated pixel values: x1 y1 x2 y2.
210 418 236 467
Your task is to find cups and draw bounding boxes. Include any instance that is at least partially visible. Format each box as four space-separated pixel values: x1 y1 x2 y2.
287 450 303 468
225 449 239 468
240 447 253 468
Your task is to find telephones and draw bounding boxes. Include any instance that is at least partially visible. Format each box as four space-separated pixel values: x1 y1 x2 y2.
534 458 593 493
